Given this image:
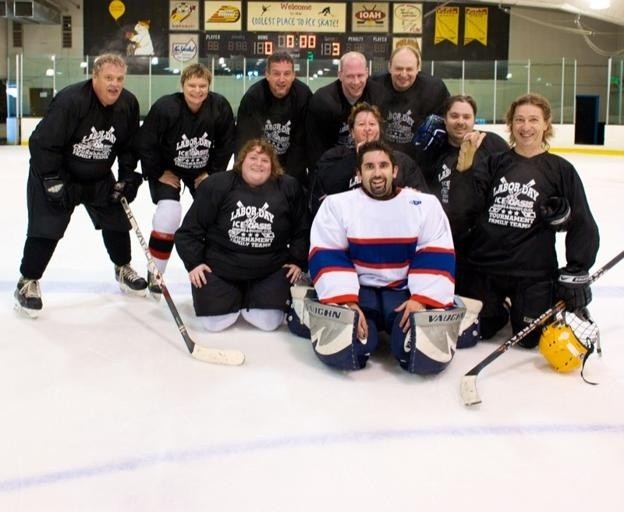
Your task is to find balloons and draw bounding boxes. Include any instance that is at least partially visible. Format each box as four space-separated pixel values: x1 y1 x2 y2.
107 0 126 20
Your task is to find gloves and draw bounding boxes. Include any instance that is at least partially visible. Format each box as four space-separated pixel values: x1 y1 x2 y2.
111 170 142 207
555 273 592 309
538 197 571 229
43 176 70 214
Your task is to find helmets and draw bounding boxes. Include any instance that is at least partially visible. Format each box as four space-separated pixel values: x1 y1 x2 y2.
539 312 597 373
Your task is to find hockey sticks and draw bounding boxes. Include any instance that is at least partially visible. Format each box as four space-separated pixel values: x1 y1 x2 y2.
120 196 245 365
461 252 622 406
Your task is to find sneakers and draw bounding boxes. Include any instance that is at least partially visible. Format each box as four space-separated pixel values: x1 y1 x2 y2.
476 308 511 340
14 279 41 309
114 266 148 288
148 271 163 294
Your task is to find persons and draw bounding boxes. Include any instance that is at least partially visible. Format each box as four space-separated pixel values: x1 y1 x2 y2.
461 94 600 348
309 103 427 215
14 54 148 318
234 51 314 188
366 43 452 165
141 63 236 299
308 50 388 166
174 139 314 332
419 92 511 248
285 141 467 374
